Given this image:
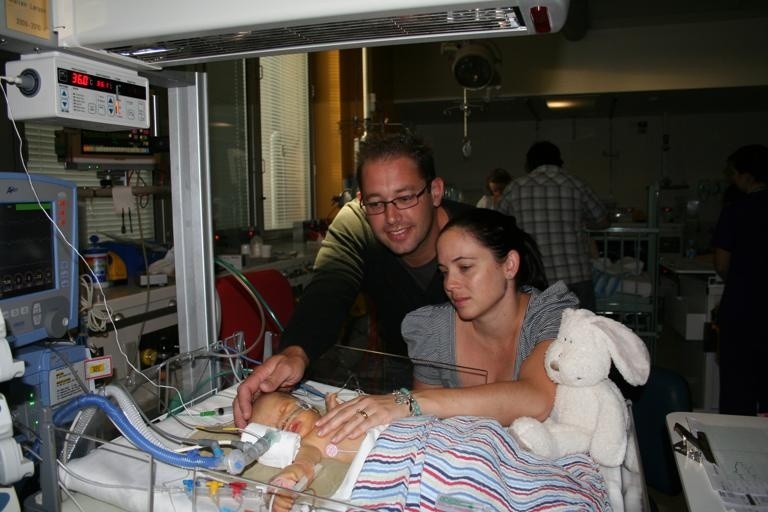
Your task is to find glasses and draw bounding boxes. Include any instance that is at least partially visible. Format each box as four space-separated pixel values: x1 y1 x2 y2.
360 185 427 215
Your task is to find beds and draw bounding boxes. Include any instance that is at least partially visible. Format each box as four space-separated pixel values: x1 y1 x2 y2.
28 328 685 511
577 226 664 348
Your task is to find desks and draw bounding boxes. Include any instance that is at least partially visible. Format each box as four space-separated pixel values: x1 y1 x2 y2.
660 408 768 512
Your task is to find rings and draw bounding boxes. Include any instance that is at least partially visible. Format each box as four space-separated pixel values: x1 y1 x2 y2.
356 407 373 422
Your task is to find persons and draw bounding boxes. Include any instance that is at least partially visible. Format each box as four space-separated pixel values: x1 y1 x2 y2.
474 167 514 211
231 125 479 431
313 206 583 446
245 390 368 512
493 140 611 315
710 143 768 418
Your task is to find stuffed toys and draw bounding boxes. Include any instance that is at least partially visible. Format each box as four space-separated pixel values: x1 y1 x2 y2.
510 306 653 469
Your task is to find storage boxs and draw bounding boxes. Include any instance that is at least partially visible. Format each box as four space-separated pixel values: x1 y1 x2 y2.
215 254 244 272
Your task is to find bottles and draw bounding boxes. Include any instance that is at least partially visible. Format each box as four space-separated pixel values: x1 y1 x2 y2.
81 235 112 290
250 236 264 256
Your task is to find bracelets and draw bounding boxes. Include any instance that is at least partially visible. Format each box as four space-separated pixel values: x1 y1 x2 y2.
392 385 422 418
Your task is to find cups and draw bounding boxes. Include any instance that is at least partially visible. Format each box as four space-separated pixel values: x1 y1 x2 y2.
261 244 272 258
240 244 250 255
